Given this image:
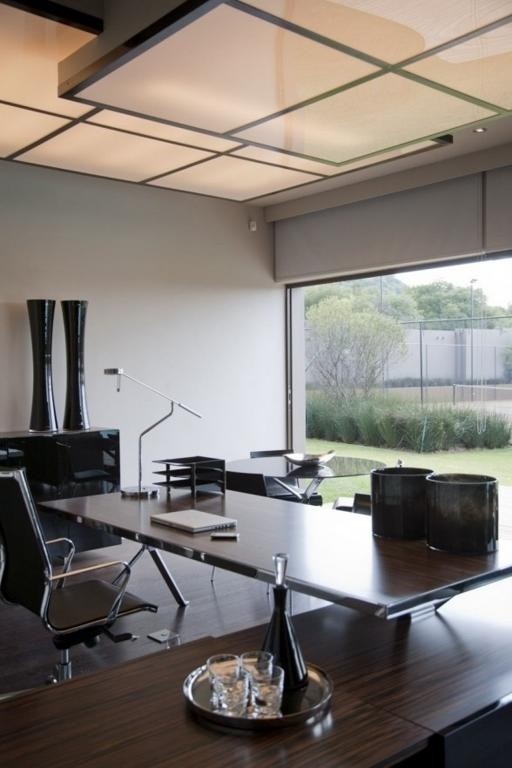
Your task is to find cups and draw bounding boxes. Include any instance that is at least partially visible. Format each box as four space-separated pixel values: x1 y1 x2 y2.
206 650 285 716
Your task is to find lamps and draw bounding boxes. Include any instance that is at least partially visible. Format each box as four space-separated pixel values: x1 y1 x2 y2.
105 368 202 494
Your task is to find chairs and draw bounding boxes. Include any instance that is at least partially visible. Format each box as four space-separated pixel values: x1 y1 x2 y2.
0 467 158 681
250 450 314 503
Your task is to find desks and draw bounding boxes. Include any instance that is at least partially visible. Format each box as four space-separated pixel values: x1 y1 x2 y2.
225 453 385 509
39 482 512 619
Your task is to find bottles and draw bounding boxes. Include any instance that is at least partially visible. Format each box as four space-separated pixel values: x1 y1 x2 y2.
257 552 309 690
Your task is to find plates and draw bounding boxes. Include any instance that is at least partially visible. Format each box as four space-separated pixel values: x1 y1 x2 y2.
181 656 335 732
282 450 337 467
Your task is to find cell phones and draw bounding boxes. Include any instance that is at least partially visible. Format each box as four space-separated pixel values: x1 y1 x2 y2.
212 533 238 539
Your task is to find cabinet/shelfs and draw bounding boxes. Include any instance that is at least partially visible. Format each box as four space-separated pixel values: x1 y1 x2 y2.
1 427 120 549
0 599 512 766
